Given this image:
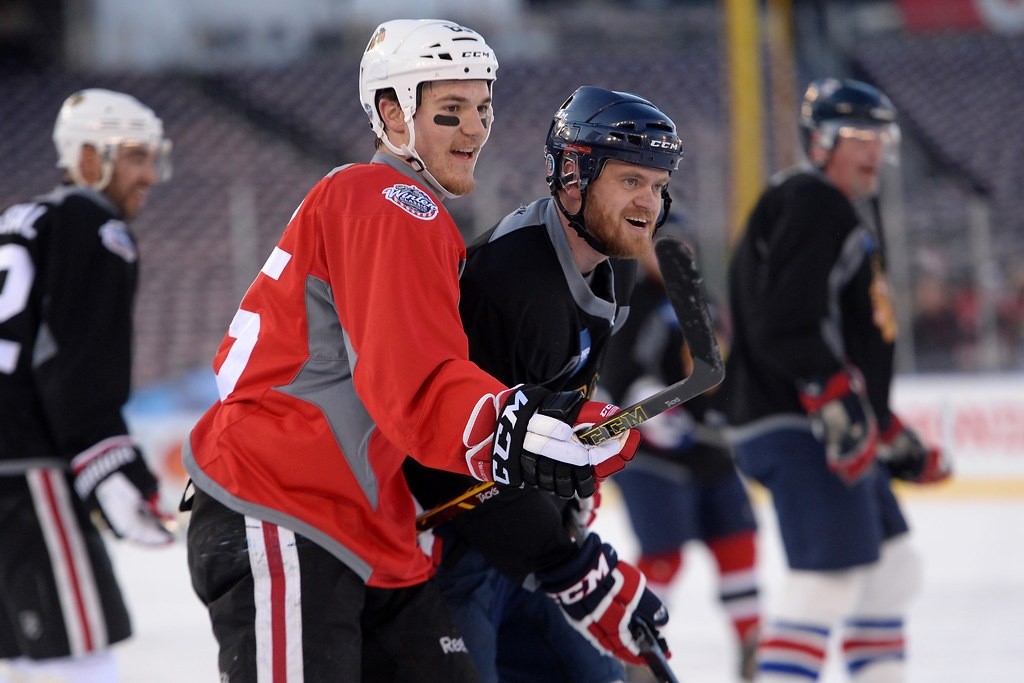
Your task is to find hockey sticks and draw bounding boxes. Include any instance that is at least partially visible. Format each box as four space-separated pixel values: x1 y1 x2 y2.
413 232 728 529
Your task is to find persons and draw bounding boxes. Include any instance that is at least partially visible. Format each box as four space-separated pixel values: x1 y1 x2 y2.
591 189 762 680
0 88 175 681
717 76 953 682
400 86 679 682
180 18 642 683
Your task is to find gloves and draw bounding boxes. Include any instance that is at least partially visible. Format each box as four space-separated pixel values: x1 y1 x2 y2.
875 413 952 484
492 384 641 500
540 532 672 665
74 445 176 547
800 365 880 484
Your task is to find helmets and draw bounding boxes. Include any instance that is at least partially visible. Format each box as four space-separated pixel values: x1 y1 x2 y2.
800 76 896 172
543 85 683 196
359 18 499 137
51 87 164 192
